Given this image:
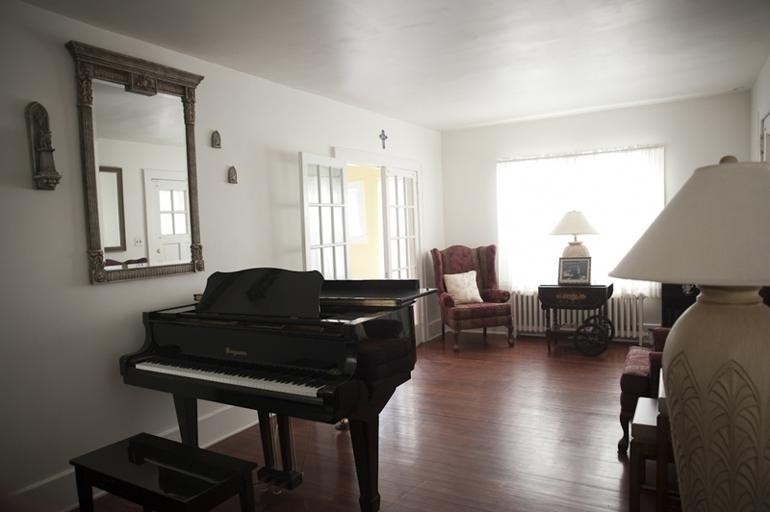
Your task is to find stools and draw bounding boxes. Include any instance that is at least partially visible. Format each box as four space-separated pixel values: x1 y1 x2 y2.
69 430 259 511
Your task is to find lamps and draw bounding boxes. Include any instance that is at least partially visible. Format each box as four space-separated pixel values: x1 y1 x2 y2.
549 207 597 257
608 160 769 512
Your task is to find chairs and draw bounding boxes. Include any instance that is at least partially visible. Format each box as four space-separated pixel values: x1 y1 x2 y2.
616 327 670 457
426 244 515 352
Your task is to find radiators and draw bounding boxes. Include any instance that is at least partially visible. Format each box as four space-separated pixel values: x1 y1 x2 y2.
511 289 647 345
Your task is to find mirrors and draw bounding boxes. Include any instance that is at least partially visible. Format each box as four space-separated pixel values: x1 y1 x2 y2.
66 39 204 285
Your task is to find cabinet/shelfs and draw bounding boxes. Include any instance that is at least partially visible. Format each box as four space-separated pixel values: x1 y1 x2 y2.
628 367 676 512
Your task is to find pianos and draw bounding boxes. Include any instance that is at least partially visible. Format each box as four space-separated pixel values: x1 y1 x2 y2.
119 268 419 512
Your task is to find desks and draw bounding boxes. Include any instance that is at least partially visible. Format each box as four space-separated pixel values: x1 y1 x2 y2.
539 283 615 357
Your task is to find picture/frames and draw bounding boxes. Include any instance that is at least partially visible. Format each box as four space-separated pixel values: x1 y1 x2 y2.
558 257 591 285
